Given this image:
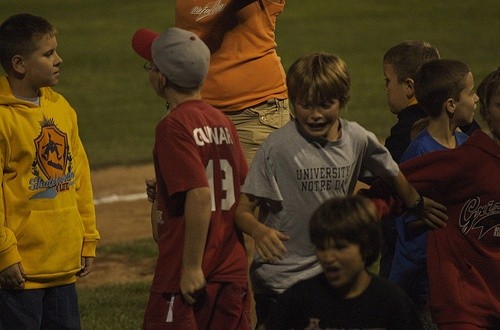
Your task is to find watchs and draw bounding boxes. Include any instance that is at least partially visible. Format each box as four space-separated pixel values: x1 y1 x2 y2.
402 193 425 214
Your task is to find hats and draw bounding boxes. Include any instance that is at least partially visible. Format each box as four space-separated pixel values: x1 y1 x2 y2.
132 27 210 89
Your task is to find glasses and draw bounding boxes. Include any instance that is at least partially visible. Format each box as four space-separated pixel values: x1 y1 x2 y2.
143 61 157 72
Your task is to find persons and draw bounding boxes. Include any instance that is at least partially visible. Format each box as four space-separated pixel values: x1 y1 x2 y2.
389 60 479 330
0 13 101 330
261 195 436 330
356 40 442 330
131 25 250 330
151 0 289 330
233 53 448 330
356 69 500 330
